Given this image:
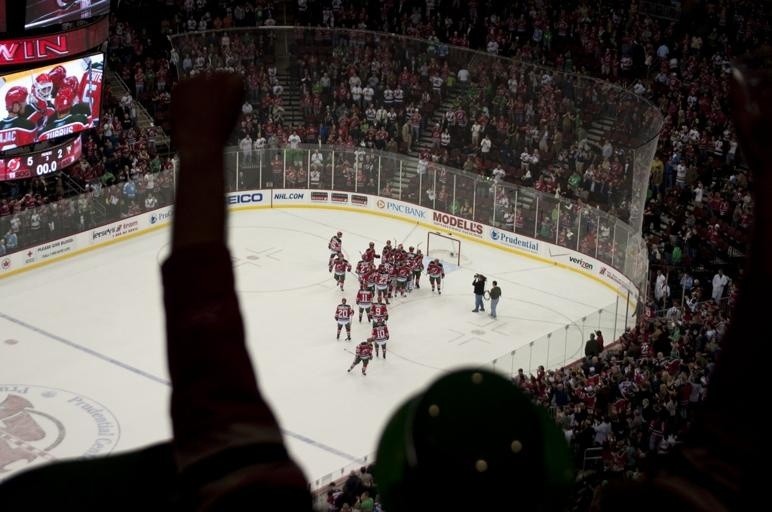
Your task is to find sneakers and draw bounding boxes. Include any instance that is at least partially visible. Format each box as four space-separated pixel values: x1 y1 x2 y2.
386 301 391 305
348 366 367 375
386 294 406 298
432 285 442 294
416 282 419 287
359 316 371 323
337 334 351 340
472 308 484 312
376 351 386 359
337 281 344 291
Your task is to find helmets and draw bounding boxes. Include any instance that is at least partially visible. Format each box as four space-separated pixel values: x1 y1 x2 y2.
337 232 343 238
338 253 344 261
370 241 374 248
384 240 414 252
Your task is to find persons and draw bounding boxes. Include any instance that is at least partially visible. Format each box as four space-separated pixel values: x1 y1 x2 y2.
146 59 772 511
1 1 769 257
323 258 743 511
